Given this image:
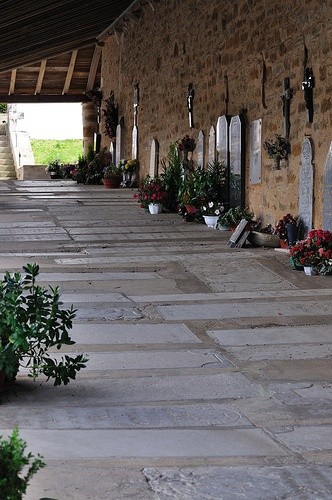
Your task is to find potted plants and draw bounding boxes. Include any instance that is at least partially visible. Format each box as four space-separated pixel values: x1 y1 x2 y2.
102 163 119 188
0 262 90 394
219 205 255 235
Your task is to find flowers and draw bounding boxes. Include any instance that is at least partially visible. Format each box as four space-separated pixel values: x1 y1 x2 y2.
43 134 332 276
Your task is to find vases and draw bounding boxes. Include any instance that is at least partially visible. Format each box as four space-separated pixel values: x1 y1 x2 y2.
148 203 163 215
289 256 304 271
285 223 298 246
51 172 56 175
303 266 317 276
203 215 220 228
279 238 289 248
217 220 231 231
